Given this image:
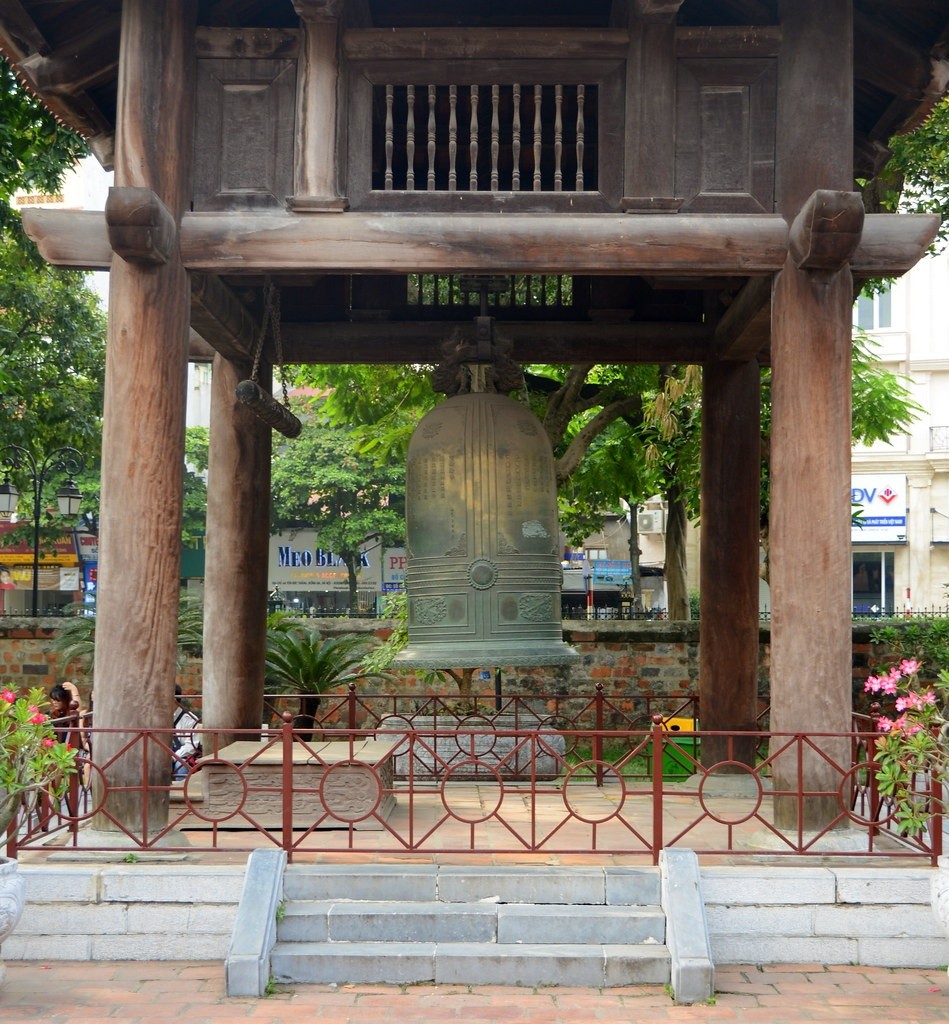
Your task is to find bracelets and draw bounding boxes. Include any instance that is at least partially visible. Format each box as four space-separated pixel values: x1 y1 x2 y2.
72 693 80 697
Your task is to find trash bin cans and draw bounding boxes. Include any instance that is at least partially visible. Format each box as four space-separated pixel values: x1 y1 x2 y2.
649 717 699 783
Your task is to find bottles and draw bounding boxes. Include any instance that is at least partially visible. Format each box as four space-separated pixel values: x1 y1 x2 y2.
176 756 190 781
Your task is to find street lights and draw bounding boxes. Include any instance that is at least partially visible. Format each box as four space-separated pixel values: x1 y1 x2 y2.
0 444 84 617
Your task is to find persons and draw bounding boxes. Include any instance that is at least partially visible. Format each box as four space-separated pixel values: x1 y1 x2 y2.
0 565 16 590
44 680 83 763
171 681 202 781
80 689 95 786
